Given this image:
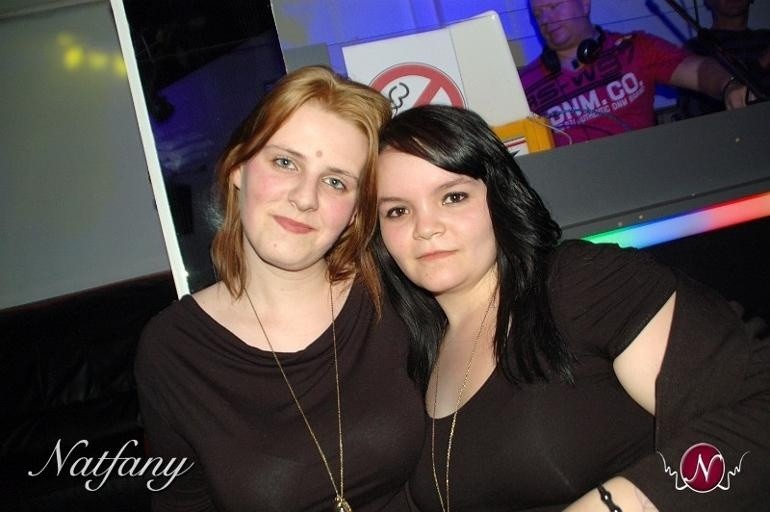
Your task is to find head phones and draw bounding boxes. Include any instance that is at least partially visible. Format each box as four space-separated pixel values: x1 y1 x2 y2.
540 23 605 73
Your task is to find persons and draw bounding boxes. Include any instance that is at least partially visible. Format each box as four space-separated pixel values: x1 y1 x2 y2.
132 65 424 512
517 0 757 148
675 0 770 120
368 104 770 512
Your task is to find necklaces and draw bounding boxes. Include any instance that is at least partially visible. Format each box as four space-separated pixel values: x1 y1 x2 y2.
431 300 491 512
240 272 353 512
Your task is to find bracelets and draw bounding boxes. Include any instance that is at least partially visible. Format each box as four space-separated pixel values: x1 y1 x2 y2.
722 76 735 107
592 479 623 512
